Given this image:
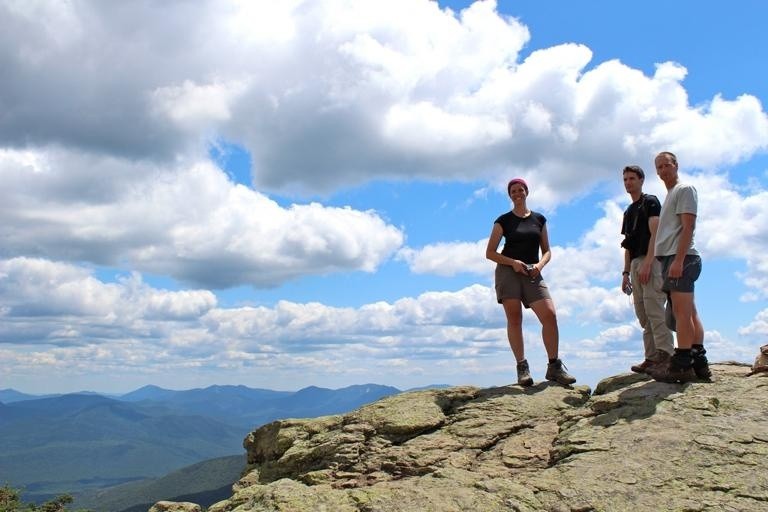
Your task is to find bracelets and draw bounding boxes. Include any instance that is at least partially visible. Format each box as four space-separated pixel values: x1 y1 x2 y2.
623 271 630 275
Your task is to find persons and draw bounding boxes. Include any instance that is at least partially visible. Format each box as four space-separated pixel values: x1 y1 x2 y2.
486 179 577 385
622 165 675 381
654 151 712 383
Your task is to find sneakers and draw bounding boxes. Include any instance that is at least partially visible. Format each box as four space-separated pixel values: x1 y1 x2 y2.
546 360 576 384
517 360 533 386
631 349 710 383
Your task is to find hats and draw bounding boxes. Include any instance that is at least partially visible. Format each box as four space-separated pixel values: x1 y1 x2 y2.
508 179 528 196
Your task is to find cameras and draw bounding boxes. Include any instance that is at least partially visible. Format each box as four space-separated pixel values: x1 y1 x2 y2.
525 264 533 271
623 284 632 295
